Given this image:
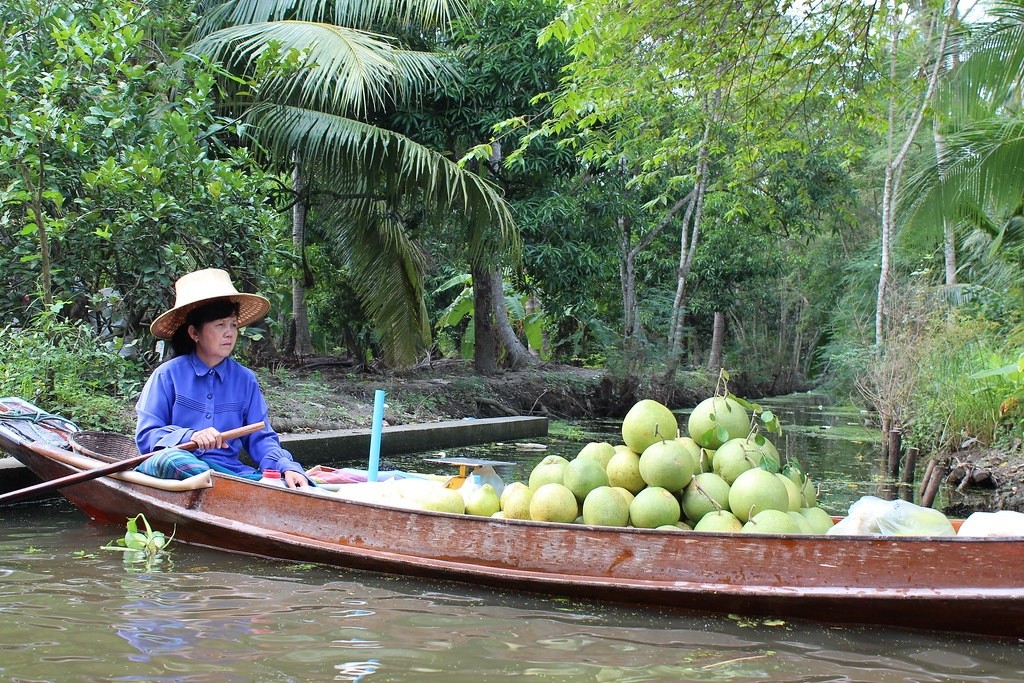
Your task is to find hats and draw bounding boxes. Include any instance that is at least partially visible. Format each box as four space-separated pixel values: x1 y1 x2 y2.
150 269 270 340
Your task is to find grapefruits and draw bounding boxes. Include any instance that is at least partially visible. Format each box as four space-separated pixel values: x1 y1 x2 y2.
424 369 834 534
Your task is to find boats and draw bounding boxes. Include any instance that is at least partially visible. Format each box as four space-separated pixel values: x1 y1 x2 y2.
0 397 1024 632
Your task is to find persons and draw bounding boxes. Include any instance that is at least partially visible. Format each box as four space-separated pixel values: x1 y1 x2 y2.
134 269 315 493
